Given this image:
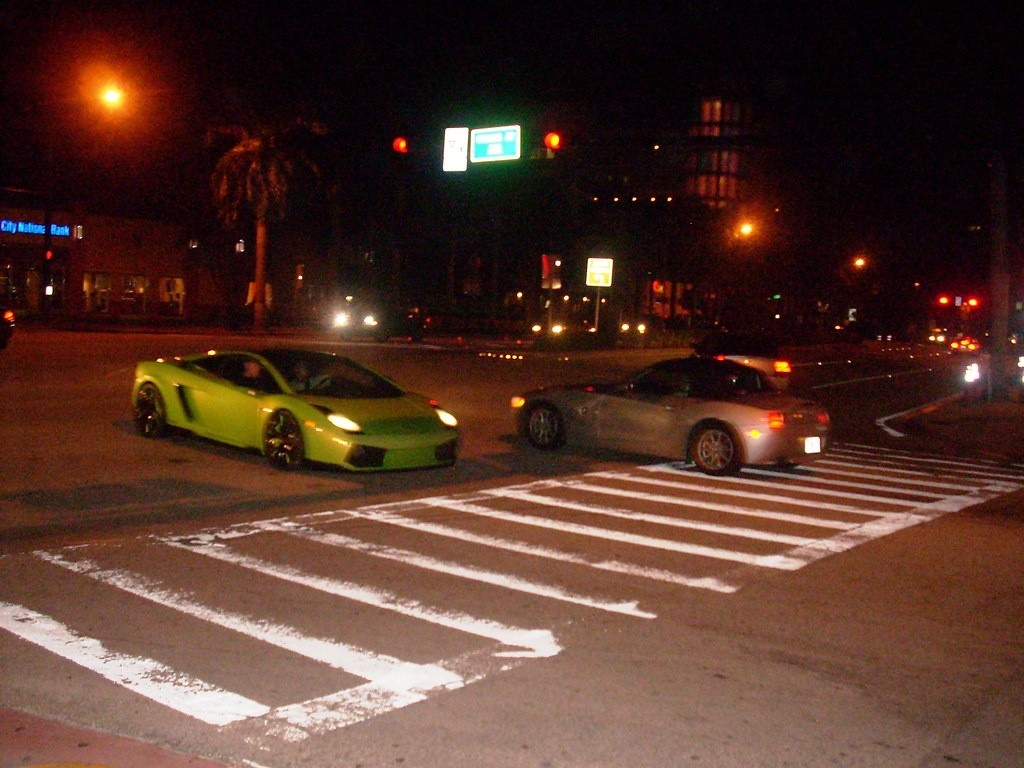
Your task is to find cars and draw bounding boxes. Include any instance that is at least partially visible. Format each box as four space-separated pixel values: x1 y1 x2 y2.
511 351 833 477
690 331 790 388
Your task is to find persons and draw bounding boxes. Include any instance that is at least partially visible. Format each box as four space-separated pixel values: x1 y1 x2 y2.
288 360 308 390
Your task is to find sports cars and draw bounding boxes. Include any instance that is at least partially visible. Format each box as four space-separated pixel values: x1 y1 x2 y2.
128 347 461 474
